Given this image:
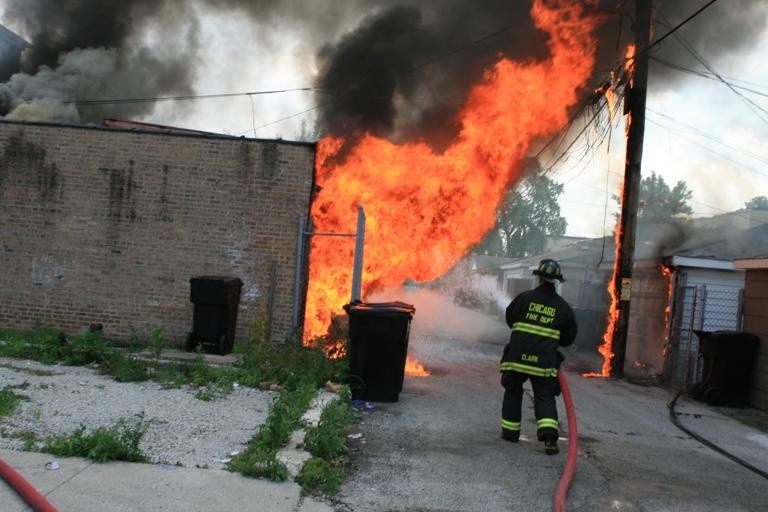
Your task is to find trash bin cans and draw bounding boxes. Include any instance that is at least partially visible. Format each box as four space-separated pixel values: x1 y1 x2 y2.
688 330 761 407
185 276 244 355
343 300 416 402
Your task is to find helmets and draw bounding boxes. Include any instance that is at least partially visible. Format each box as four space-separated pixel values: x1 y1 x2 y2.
532 260 565 282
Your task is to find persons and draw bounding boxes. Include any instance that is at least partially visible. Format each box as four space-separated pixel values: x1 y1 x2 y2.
499 259 578 455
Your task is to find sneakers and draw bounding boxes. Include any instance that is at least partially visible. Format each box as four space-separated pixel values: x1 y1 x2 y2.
544 431 559 454
503 434 518 442
